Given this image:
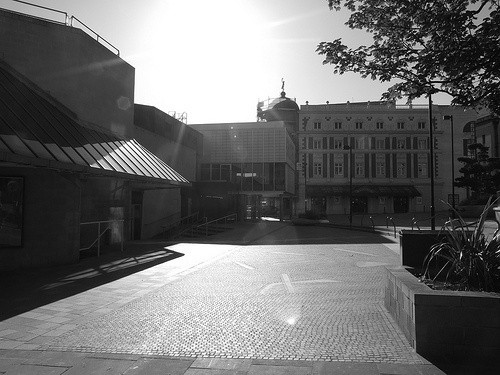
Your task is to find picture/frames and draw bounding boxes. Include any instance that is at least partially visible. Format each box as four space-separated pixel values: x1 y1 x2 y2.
0 175 25 249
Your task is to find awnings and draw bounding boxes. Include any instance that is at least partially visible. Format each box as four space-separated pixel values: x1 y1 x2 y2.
0 70 192 189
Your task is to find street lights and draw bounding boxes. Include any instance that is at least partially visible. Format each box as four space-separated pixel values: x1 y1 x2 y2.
443 115 455 219
418 86 436 231
343 145 353 225
